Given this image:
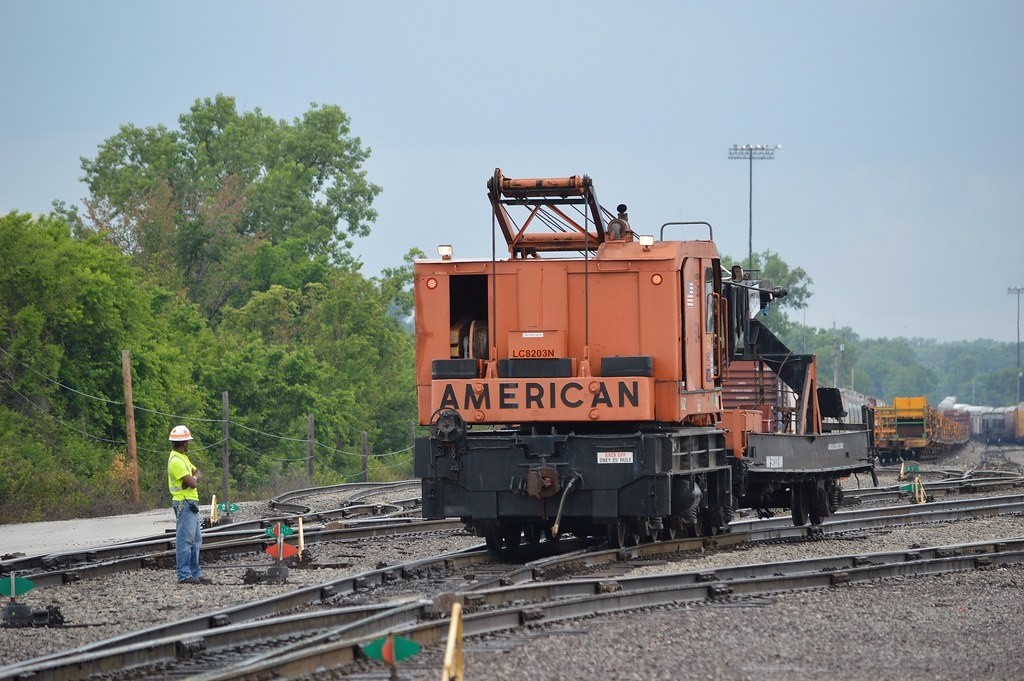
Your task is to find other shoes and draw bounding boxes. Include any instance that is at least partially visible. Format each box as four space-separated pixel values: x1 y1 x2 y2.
199 576 213 583
177 577 200 584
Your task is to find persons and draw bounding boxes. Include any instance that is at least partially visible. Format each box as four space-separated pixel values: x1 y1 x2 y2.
167 425 213 584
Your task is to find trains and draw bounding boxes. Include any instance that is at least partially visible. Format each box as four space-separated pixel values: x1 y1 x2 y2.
867 396 1024 465
414 169 875 550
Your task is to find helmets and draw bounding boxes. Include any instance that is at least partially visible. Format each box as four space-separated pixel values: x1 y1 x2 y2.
168 425 194 441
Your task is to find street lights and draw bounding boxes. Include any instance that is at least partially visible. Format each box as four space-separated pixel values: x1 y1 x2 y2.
1008 287 1024 402
728 144 781 278
